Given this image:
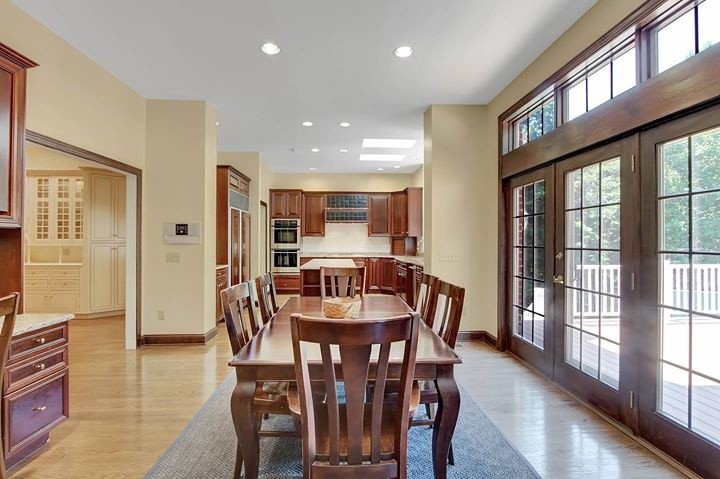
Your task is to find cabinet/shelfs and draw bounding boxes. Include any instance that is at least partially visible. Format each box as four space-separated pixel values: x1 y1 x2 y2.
24 267 80 313
268 189 327 237
273 257 422 295
5 322 69 479
216 267 228 322
0 38 41 227
366 187 422 237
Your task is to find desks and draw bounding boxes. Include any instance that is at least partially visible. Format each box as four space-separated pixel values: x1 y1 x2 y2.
299 259 356 296
227 293 463 479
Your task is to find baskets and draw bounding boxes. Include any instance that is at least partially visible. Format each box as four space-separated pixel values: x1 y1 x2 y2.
322 300 362 318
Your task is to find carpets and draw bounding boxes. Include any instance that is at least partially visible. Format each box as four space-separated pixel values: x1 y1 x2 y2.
144 369 543 479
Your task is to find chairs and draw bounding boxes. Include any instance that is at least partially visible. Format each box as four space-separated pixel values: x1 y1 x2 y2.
219 265 466 479
